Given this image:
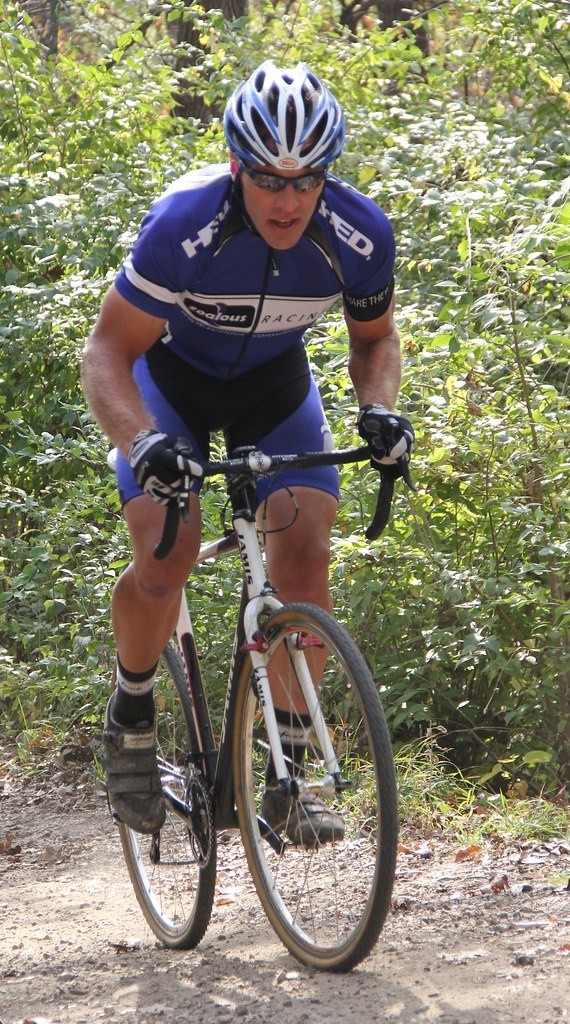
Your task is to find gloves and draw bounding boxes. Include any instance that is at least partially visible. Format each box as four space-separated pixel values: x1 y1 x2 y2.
130 429 204 510
356 401 415 477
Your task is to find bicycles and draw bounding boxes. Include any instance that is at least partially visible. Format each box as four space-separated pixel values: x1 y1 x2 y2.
107 399 417 975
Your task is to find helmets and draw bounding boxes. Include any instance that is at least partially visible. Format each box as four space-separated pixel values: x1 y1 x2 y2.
223 58 346 170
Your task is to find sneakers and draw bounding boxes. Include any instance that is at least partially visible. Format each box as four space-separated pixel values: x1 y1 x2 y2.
103 690 166 835
262 779 346 849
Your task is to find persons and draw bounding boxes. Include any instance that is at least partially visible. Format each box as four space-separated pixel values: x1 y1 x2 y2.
81 60 417 846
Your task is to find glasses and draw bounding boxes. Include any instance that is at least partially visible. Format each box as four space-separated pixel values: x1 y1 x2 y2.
237 154 326 192
